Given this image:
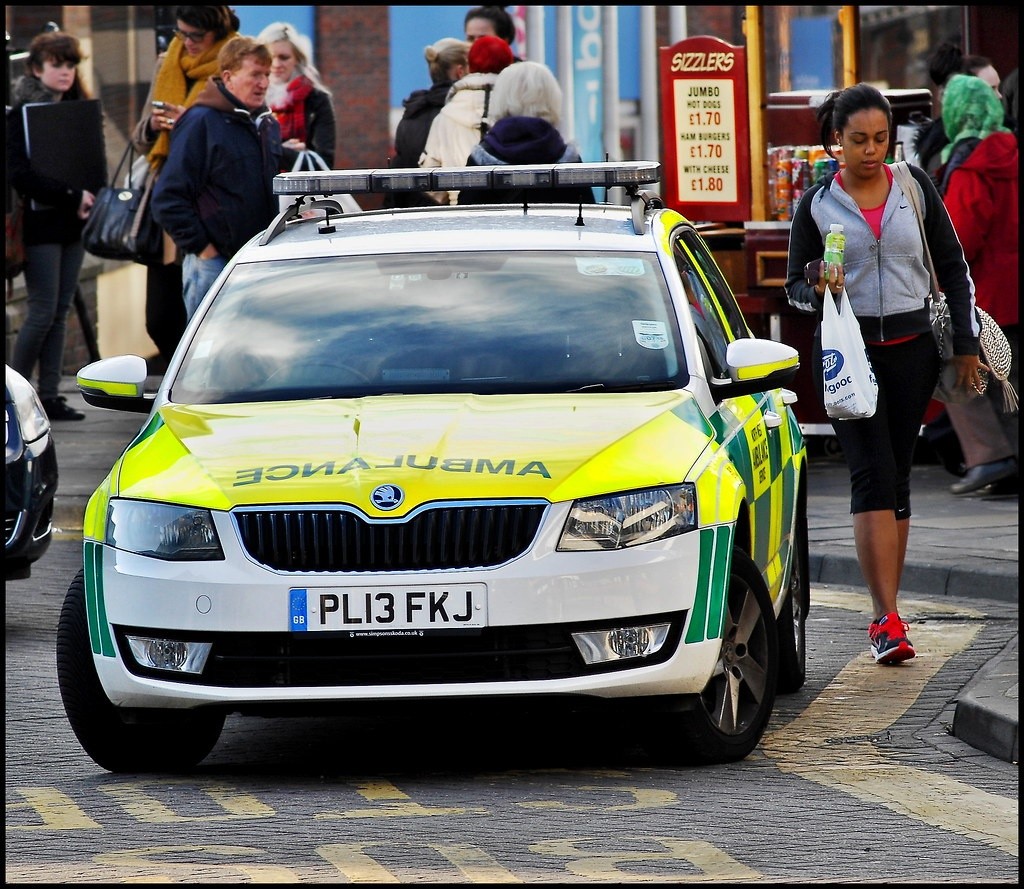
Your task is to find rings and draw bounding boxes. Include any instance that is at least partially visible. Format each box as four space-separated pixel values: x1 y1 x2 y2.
168 119 174 124
837 285 842 289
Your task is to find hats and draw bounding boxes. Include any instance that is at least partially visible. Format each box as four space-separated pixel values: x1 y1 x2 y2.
468 35 516 75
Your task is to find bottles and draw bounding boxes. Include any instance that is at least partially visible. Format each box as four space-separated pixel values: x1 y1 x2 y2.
825 224 845 281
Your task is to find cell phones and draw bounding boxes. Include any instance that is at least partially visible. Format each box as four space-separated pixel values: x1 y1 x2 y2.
151 99 166 107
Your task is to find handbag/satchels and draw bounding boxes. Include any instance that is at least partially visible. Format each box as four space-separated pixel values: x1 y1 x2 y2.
81 140 160 259
278 149 365 219
820 282 879 422
927 291 1019 413
2 197 26 279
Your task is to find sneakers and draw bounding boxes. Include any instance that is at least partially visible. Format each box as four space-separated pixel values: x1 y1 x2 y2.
867 608 915 663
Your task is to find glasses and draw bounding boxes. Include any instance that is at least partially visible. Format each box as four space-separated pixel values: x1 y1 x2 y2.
172 26 209 43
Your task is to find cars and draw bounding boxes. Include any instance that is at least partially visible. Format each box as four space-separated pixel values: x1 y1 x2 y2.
5 363 60 581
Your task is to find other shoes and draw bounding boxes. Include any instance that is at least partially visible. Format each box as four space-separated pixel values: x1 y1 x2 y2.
950 455 1020 501
39 395 86 421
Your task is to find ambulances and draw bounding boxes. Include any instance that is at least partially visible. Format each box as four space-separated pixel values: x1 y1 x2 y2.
57 159 812 777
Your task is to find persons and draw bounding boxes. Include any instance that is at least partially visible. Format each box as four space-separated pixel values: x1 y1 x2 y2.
7 5 601 421
783 84 990 662
915 44 1020 497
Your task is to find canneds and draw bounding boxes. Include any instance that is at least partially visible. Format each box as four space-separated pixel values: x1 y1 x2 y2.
768 146 843 221
